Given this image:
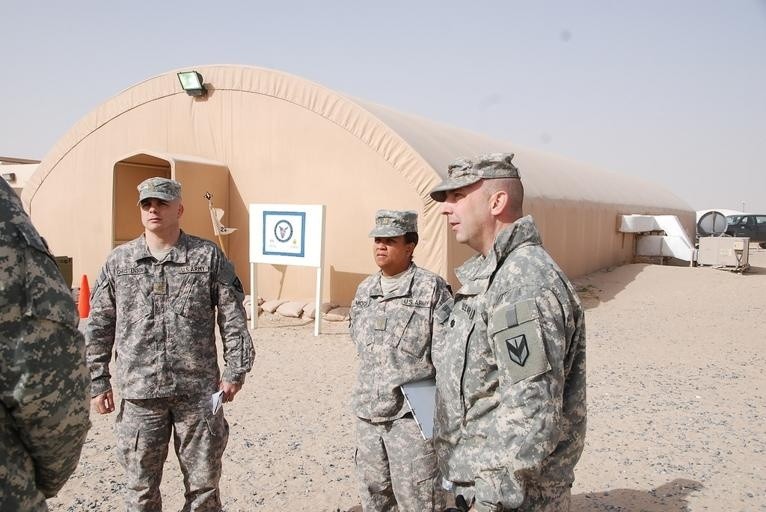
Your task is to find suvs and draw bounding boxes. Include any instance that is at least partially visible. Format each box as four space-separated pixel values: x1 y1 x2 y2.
726 215 766 248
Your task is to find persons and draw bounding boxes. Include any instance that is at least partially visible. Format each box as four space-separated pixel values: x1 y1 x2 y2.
348 208 454 512
84 177 256 512
1 177 92 512
429 151 587 512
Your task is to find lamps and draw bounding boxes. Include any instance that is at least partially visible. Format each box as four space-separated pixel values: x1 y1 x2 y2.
177 71 205 96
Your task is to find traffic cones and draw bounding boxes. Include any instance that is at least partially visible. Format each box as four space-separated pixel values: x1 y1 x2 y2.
77 275 90 318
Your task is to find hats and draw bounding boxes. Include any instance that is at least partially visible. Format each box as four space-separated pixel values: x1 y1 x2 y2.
368 207 423 240
429 151 521 204
134 173 182 209
